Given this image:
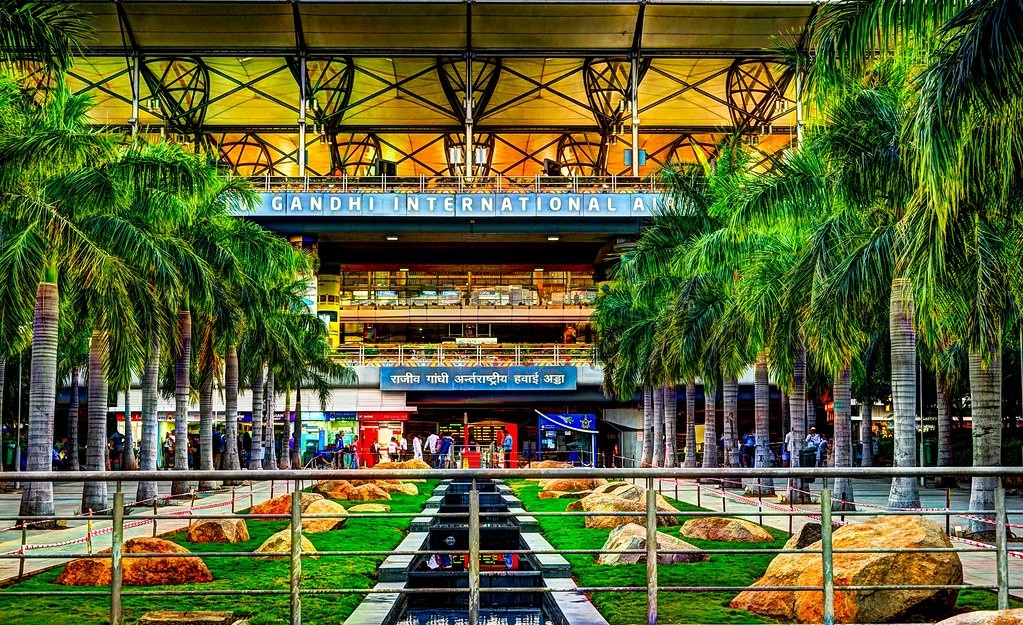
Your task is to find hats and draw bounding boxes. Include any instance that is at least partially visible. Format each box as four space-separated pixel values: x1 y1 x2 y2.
811 427 816 430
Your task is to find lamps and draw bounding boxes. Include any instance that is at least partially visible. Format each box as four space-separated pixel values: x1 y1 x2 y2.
462 100 466 109
471 100 476 108
321 124 326 135
746 136 751 145
605 136 610 145
320 135 325 144
612 136 617 145
781 101 788 113
306 99 311 112
767 124 773 135
753 137 758 146
611 125 616 135
327 135 332 144
619 100 624 112
312 98 318 111
774 101 780 112
619 125 625 136
758 125 765 136
627 100 632 112
313 124 318 135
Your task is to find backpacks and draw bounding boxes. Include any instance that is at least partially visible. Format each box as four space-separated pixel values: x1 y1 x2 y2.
434 439 442 453
440 438 454 454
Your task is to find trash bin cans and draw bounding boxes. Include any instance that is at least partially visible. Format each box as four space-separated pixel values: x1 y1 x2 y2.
78 447 87 466
800 450 817 469
924 438 938 463
2 440 17 465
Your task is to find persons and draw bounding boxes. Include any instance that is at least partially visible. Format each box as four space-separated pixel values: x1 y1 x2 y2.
335 165 343 176
468 427 477 451
662 427 879 467
497 426 513 468
20 430 454 470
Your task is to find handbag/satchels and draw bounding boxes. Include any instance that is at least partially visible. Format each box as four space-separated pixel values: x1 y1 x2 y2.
167 436 176 448
